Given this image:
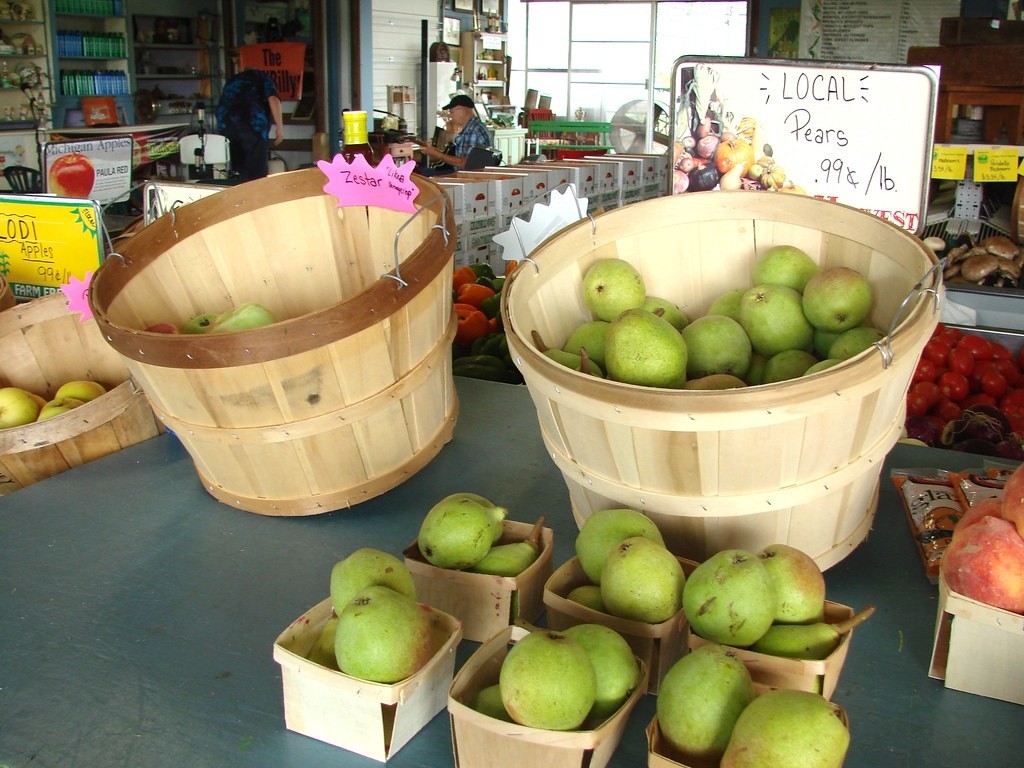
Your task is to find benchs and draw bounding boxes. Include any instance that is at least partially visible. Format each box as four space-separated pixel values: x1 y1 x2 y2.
526 121 615 157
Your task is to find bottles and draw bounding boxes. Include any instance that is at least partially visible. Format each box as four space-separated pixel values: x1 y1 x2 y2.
191 148 205 180
56 29 125 58
196 102 209 134
343 111 374 167
60 68 129 95
54 0 114 16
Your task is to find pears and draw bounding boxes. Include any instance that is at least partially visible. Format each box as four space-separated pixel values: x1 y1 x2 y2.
304 491 877 768
532 242 886 390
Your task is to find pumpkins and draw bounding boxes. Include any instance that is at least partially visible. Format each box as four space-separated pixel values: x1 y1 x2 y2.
686 118 804 195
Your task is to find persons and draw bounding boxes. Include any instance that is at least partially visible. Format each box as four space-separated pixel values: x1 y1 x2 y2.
215 68 283 178
413 95 494 178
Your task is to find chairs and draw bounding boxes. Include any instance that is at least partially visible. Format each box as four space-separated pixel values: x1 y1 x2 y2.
2 165 41 193
524 107 569 158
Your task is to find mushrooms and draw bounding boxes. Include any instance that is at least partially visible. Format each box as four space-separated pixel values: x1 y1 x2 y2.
924 234 1024 292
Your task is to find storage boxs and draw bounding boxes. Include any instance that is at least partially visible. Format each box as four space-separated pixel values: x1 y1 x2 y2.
430 154 668 277
271 517 1024 768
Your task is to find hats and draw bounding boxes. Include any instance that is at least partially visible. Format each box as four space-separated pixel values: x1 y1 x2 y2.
442 95 474 110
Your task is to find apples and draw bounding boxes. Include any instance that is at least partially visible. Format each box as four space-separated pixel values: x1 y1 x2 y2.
49 154 94 199
141 302 278 334
0 380 106 431
669 116 732 194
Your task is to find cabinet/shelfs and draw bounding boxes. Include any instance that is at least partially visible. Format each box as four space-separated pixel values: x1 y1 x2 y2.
387 85 417 139
489 128 529 166
460 30 515 114
0 0 313 130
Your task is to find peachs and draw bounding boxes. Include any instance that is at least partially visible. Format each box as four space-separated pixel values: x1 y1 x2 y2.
940 461 1024 616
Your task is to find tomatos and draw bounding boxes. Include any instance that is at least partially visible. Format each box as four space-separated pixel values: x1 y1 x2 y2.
906 321 1024 459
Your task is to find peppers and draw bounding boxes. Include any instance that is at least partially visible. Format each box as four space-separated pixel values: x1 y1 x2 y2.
452 259 518 384
